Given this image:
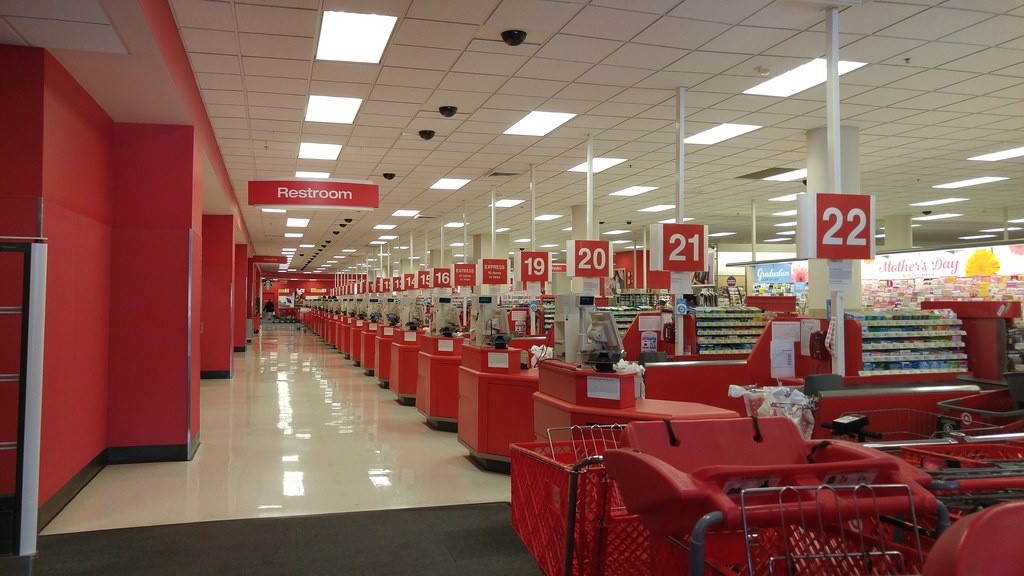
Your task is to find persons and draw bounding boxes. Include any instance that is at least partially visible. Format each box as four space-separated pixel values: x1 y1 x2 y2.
263 299 274 320
608 271 624 289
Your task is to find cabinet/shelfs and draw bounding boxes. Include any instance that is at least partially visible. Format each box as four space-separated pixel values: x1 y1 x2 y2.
389 272 1024 385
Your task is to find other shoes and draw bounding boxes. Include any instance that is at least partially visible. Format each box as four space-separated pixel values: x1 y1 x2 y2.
268 319 271 320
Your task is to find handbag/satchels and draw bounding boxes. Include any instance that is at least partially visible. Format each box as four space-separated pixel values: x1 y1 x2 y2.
273 311 275 315
271 307 274 311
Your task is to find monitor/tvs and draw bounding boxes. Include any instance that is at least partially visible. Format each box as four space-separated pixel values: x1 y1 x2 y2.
446 307 462 332
490 307 510 335
590 311 625 353
308 305 425 325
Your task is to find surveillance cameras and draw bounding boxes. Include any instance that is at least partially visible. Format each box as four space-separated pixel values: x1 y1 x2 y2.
439 106 458 117
301 219 352 270
803 180 807 185
501 30 527 46
520 248 524 251
627 221 631 224
922 211 932 215
419 130 435 140
383 173 395 180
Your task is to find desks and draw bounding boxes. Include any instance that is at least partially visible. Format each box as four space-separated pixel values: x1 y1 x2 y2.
300 306 553 466
730 375 983 444
532 391 743 445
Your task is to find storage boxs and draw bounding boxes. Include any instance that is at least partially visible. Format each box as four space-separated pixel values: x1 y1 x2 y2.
503 436 1023 574
